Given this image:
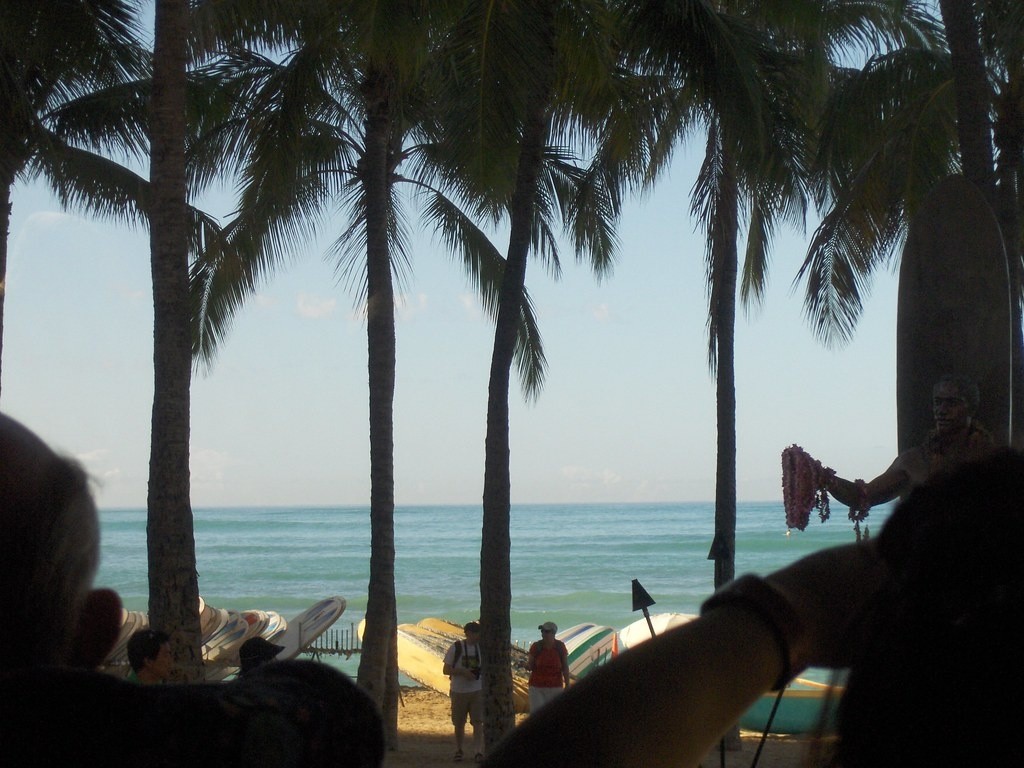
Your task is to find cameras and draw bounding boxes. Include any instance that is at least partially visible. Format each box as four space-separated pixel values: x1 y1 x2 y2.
470 670 479 680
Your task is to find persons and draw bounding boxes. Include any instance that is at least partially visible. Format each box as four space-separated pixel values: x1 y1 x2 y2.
783 376 1001 514
518 621 570 716
122 628 175 687
483 449 1024 767
233 635 287 686
0 415 387 768
443 622 483 763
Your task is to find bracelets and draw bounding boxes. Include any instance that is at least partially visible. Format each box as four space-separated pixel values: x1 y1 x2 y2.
701 575 812 689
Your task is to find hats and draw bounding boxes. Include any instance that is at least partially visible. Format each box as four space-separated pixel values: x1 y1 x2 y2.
538 621 558 634
464 621 481 633
234 637 286 675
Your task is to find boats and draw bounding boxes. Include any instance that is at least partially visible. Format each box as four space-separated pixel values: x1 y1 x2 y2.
357 618 580 713
622 613 845 735
556 622 615 681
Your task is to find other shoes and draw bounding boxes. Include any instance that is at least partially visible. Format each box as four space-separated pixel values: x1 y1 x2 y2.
474 753 485 763
454 752 465 761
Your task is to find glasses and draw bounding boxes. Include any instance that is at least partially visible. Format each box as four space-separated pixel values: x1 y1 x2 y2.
541 629 552 633
